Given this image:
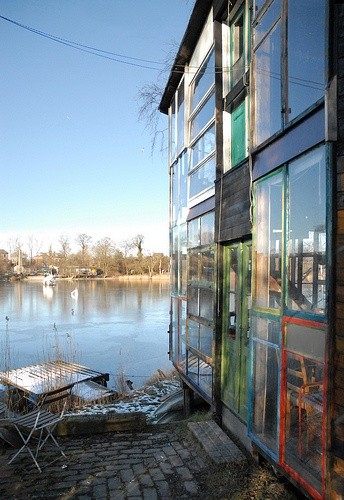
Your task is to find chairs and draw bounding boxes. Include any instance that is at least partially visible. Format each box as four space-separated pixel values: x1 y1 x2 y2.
7 388 70 473
282 351 319 454
